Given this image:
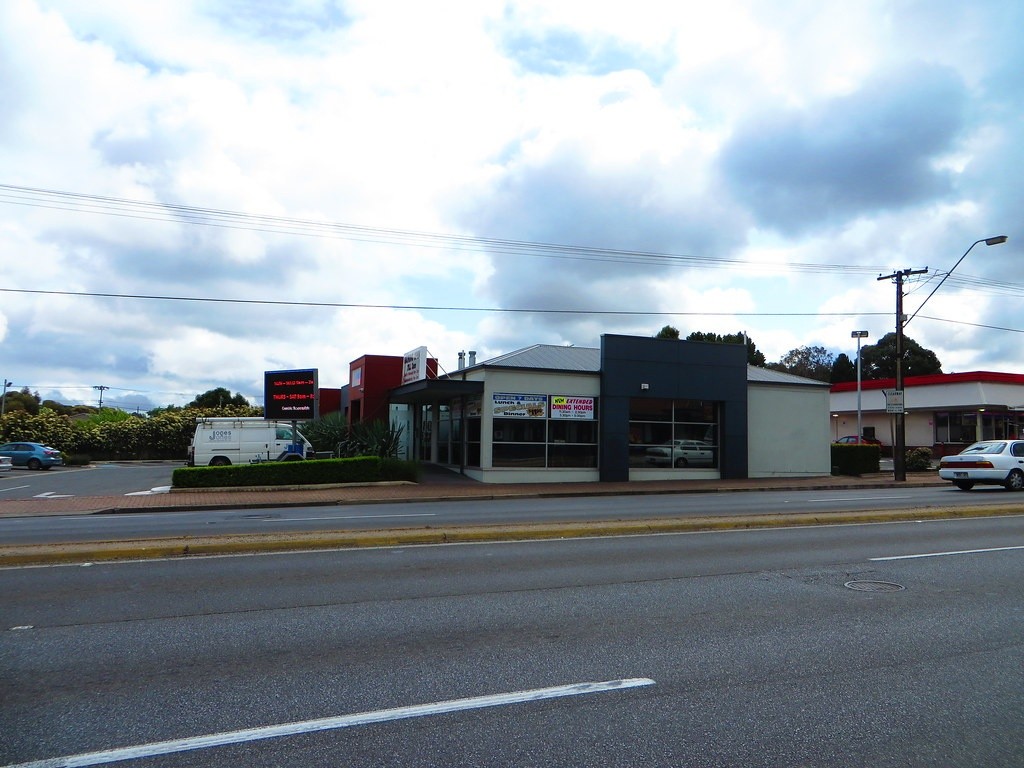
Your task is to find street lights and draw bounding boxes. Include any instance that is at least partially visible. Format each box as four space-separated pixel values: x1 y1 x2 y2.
894 235 1009 481
851 330 869 445
2 380 12 421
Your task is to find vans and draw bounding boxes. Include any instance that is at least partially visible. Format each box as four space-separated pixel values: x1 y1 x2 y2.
185 421 316 468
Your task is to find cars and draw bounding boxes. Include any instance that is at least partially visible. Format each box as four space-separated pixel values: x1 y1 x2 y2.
939 438 1024 492
645 438 714 468
0 441 63 471
834 434 882 451
